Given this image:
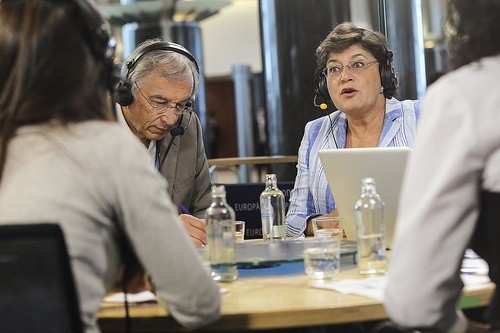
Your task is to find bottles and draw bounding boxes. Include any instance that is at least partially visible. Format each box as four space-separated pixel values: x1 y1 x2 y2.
353 176 386 274
203 185 239 283
259 174 286 243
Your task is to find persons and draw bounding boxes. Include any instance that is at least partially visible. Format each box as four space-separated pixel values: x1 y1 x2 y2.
114 38 213 248
383 0 500 333
0 0 221 333
285 22 421 239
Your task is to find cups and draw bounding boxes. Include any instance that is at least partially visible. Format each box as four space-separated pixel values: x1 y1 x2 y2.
311 217 344 243
222 220 245 242
303 236 341 279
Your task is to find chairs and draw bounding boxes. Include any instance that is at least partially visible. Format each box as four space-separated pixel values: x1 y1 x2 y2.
0 222 86 333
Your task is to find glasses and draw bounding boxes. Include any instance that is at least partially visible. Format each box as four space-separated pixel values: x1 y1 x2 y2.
322 60 383 79
135 81 192 115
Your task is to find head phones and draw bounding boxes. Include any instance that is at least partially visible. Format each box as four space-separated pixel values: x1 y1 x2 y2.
315 28 398 99
84 0 121 92
115 41 200 113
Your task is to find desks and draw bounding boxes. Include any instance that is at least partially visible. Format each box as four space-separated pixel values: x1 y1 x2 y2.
208 155 299 183
96 235 496 333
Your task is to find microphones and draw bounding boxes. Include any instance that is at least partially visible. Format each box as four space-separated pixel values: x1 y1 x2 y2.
313 96 327 110
170 111 192 137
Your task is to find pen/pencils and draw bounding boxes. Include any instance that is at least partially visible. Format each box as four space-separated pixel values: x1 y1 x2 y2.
179 203 205 245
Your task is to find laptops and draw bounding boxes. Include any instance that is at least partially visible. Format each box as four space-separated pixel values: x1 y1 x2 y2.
318 146 411 249
